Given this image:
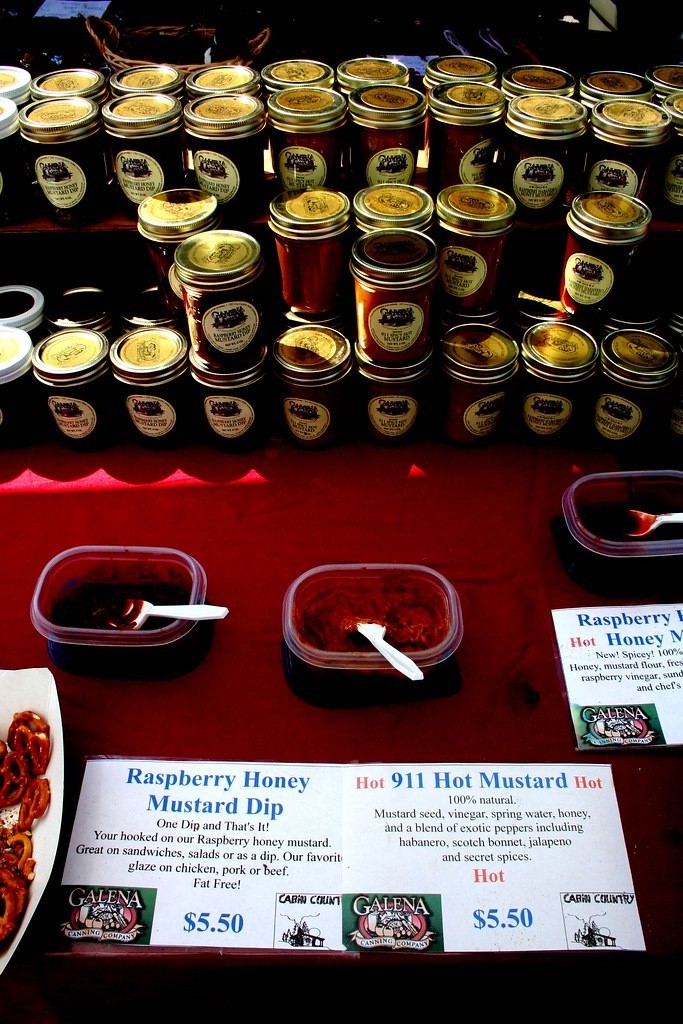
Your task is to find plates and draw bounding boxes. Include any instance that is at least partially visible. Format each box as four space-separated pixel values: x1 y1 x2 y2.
0 667 65 975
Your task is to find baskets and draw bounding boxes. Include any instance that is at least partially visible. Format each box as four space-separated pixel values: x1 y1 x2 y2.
86 15 271 76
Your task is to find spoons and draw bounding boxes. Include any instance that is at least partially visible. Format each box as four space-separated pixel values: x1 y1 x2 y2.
345 618 425 681
625 509 683 538
106 599 229 631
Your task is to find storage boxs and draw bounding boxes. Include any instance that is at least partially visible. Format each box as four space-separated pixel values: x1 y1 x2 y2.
560 470 683 601
280 563 465 711
29 545 217 683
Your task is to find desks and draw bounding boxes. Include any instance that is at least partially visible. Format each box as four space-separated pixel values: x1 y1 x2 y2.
0 444 683 985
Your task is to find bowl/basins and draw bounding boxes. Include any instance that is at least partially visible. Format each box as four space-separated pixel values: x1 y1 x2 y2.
282 562 464 709
562 470 683 588
29 543 208 675
